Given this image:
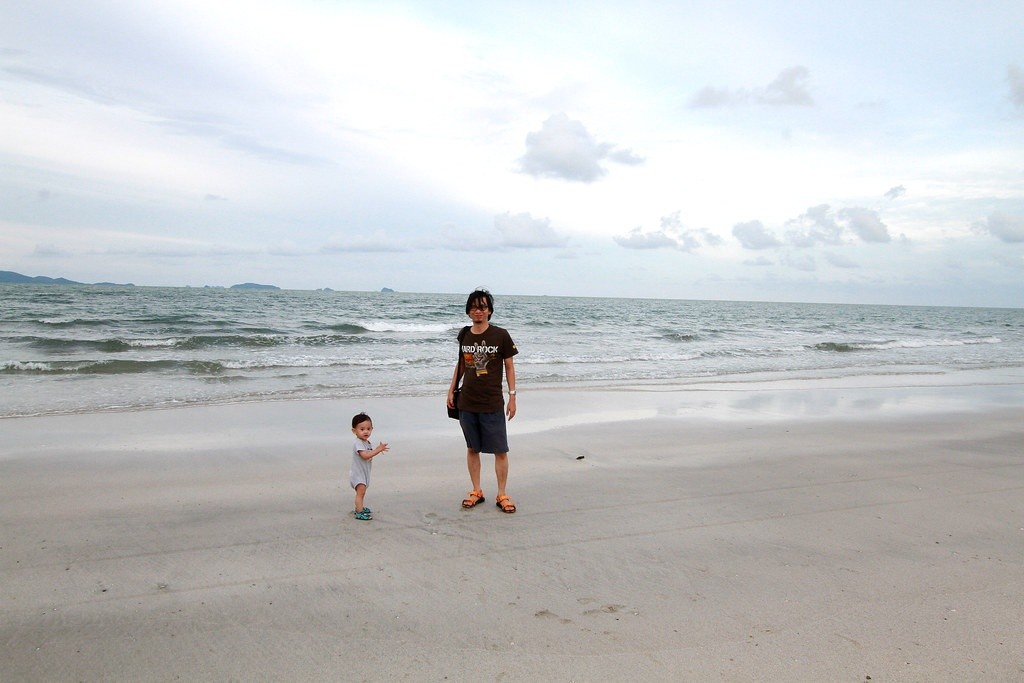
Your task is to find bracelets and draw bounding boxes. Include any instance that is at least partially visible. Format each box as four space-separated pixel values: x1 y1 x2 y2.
508 391 516 395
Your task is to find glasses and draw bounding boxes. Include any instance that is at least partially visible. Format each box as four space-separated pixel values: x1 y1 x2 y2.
470 304 488 311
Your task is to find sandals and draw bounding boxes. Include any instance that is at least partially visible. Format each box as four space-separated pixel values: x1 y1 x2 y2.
462 491 485 508
355 512 372 520
355 507 370 513
496 496 516 513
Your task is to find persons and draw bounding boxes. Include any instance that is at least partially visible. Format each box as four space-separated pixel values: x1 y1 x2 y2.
447 290 519 513
349 412 389 520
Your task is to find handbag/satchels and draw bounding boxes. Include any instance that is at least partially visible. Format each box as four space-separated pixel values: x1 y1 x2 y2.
447 391 461 419
